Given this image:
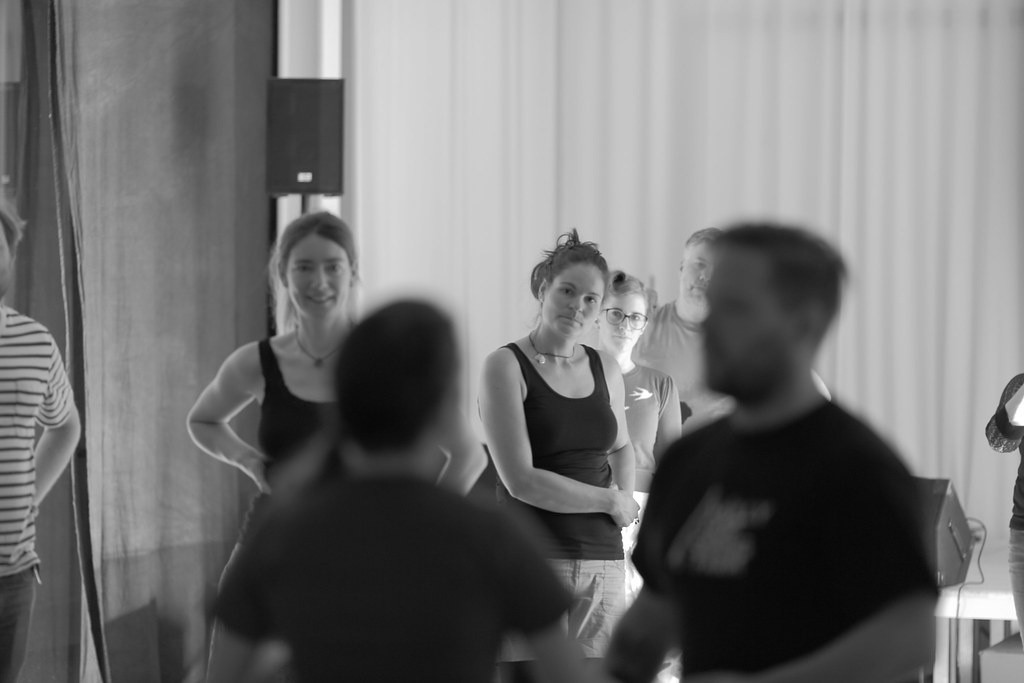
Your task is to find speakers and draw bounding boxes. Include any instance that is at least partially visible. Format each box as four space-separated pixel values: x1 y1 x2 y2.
267 78 344 197
916 476 975 588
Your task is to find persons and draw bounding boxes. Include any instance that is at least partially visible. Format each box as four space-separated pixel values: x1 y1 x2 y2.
186 211 488 683
0 203 82 683
601 226 940 682
630 226 731 418
985 373 1024 645
601 271 683 553
198 300 617 683
479 229 640 682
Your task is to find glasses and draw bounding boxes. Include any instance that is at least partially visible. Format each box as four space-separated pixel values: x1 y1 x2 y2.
601 307 648 331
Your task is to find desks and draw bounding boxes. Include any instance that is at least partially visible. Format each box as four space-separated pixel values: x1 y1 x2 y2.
921 569 1024 683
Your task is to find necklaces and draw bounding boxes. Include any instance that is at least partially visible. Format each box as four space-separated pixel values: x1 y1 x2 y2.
294 315 352 367
530 329 575 364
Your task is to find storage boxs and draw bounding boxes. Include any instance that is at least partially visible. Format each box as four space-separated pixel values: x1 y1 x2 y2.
979 632 1024 683
